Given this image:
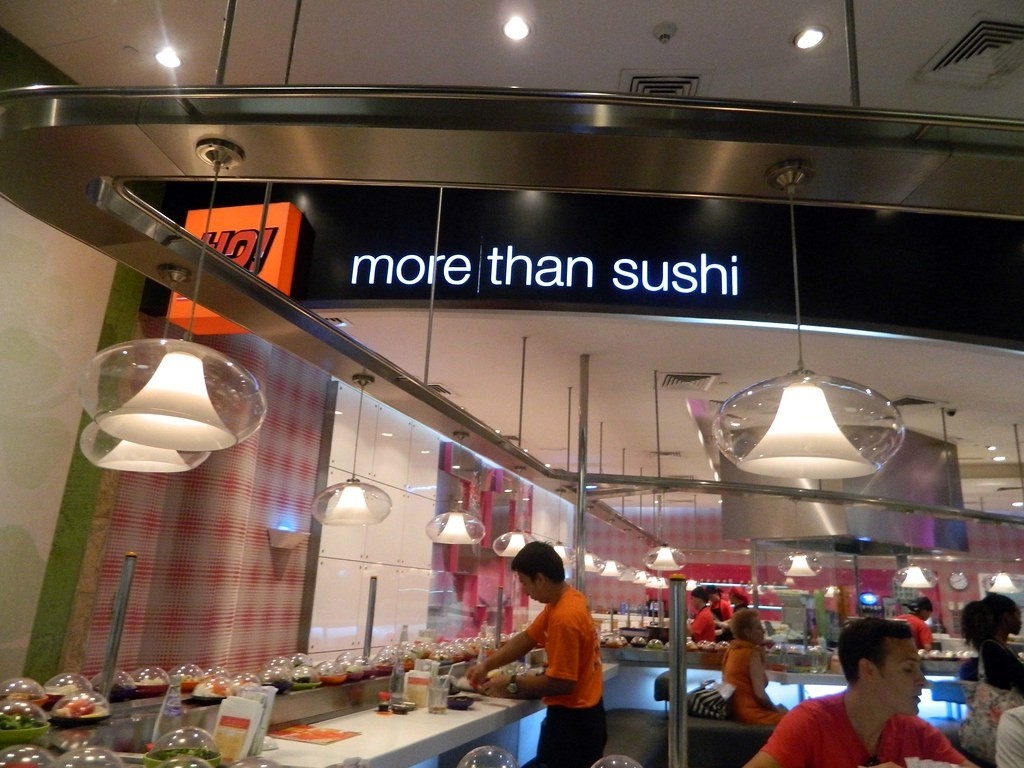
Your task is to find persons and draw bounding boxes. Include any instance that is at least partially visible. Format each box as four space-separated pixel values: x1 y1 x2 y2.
713 587 750 628
721 607 786 726
742 616 981 768
464 540 611 768
890 597 935 650
707 585 735 645
957 591 1024 768
684 587 715 643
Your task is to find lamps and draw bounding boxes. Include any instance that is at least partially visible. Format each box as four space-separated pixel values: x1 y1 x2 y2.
311 373 392 526
427 430 488 546
79 133 269 472
986 526 1020 595
777 494 822 578
712 159 904 479
266 526 312 550
492 367 685 588
891 513 939 589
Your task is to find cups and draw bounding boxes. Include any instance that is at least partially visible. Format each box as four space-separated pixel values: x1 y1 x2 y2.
427 676 450 715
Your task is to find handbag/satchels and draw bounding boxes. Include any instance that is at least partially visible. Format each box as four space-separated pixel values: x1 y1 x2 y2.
957 641 1024 765
686 680 734 721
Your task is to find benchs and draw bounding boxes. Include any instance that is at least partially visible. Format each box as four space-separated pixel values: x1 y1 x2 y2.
513 669 999 767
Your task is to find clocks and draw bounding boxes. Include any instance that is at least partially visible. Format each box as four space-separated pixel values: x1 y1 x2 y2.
948 572 968 591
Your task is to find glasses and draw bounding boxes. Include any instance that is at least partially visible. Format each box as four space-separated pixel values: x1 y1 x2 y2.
866 754 879 767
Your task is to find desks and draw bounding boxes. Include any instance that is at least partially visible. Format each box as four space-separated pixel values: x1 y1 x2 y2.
260 657 621 768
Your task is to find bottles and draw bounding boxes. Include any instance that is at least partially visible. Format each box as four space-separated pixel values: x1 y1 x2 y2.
399 624 408 641
377 691 390 712
477 646 485 665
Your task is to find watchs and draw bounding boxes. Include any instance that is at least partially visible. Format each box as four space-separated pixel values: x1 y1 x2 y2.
507 674 518 699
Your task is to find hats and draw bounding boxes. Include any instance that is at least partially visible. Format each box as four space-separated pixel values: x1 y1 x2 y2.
692 587 708 601
705 585 723 594
903 597 932 614
731 586 749 606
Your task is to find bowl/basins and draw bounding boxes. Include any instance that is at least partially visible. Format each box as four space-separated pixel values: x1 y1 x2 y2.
447 696 474 710
320 666 395 685
